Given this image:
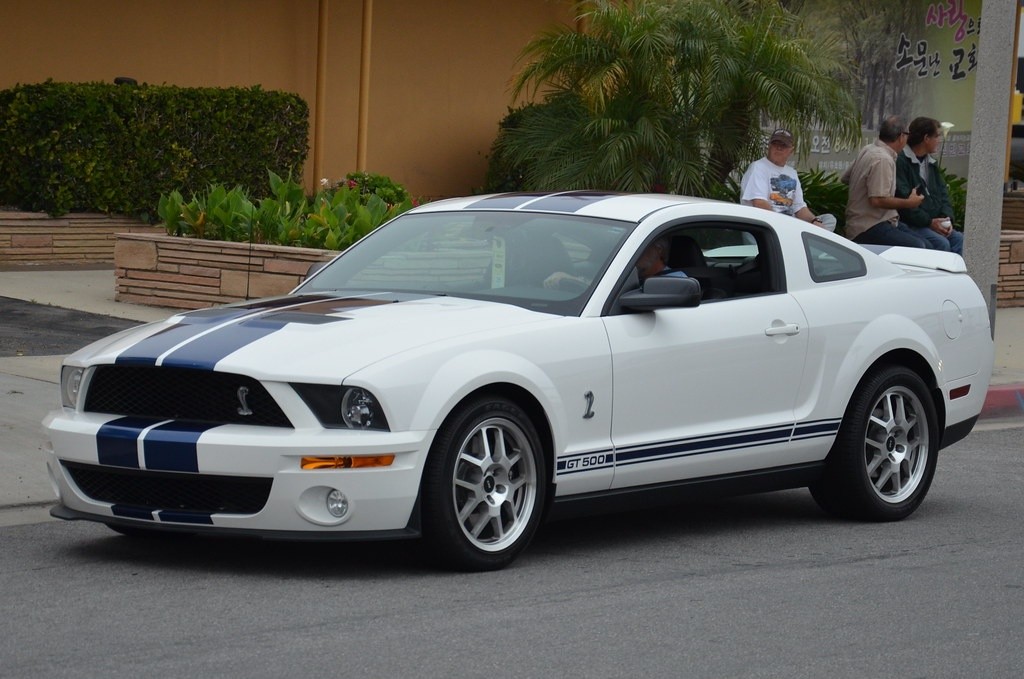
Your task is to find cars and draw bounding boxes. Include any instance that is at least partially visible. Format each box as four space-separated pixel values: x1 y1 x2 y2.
37 190 997 572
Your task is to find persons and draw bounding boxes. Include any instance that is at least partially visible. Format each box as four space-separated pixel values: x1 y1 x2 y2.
741 129 825 246
542 236 687 290
894 116 964 256
841 114 934 249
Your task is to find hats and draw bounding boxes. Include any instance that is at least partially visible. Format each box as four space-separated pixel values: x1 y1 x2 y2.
769 129 794 147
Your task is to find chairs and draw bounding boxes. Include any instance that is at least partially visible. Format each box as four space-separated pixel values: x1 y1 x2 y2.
666 236 734 299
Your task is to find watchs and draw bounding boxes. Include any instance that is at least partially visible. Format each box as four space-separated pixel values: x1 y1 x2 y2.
811 218 822 224
